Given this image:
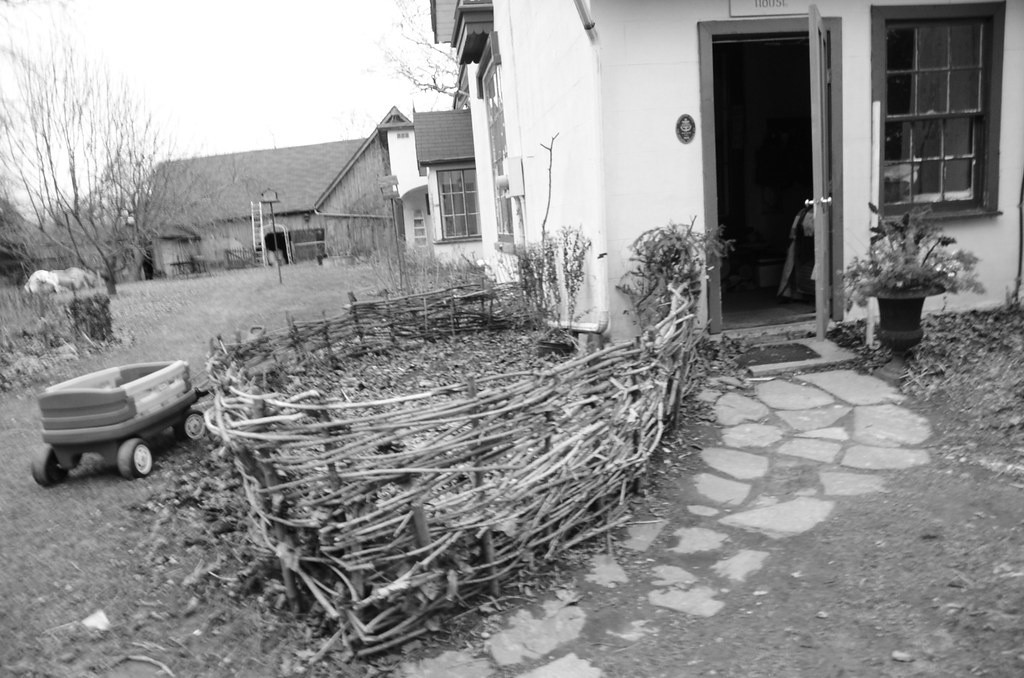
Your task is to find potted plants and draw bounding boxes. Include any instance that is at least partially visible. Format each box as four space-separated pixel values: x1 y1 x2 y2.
837 202 985 384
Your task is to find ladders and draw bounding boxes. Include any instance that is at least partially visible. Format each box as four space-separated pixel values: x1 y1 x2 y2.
411 208 431 260
250 201 267 268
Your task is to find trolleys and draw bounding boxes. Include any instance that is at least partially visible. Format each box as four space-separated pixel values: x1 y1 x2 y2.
29 325 267 489
